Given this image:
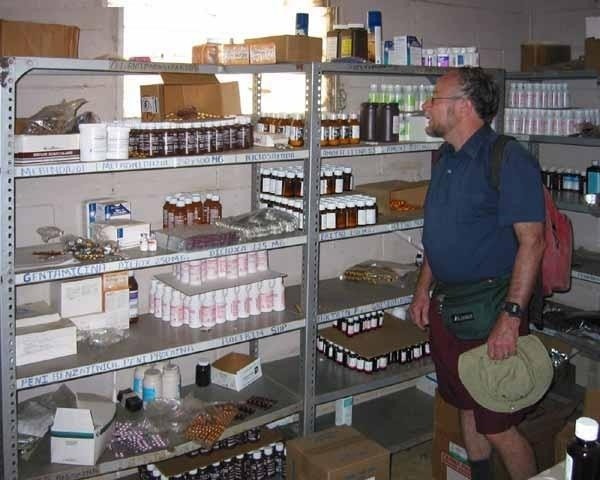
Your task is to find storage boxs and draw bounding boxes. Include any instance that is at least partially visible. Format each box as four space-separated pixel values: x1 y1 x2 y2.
0 18 80 58
244 35 322 62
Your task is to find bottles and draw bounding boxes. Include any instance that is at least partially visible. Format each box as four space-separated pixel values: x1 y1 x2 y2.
335 395 353 428
163 192 222 228
139 428 287 480
504 82 600 138
293 10 481 67
77 84 435 164
128 233 290 330
315 311 431 374
539 160 600 194
564 416 600 480
258 164 379 231
133 359 212 409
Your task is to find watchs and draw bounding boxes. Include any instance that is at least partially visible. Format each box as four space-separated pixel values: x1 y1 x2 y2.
502 300 521 319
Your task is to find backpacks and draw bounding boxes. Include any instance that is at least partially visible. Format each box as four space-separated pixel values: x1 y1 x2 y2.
487 136 573 297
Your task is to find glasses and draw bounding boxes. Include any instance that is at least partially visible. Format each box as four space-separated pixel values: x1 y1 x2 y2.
425 95 460 107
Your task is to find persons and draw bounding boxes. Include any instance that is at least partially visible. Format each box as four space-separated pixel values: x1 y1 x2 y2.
406 66 572 480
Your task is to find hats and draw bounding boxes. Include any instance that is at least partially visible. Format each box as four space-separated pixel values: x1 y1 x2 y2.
458 335 554 415
